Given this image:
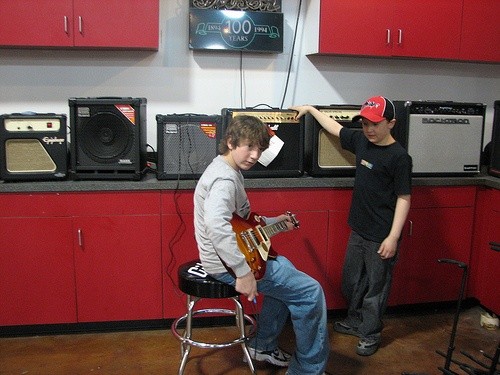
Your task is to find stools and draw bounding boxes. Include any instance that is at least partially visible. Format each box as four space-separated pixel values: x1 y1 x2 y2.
171 259 258 375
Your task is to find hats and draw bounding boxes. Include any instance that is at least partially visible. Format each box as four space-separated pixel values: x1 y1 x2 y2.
351 95 396 125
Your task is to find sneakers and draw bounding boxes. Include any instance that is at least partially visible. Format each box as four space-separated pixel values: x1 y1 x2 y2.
247 345 292 366
334 321 360 336
356 332 380 356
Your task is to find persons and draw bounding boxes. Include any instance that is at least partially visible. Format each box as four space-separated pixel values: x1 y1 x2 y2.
288 96 413 356
193 116 330 375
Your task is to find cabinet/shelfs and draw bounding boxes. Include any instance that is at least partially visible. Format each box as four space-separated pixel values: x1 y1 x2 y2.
306 0 500 65
0 176 500 338
0 0 160 51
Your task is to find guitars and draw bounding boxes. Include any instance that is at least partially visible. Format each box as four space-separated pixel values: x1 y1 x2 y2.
229 211 300 280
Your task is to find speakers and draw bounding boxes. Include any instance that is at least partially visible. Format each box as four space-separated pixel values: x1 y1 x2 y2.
0 113 67 180
305 105 365 178
156 114 223 180
222 107 306 177
68 98 147 181
393 100 488 175
